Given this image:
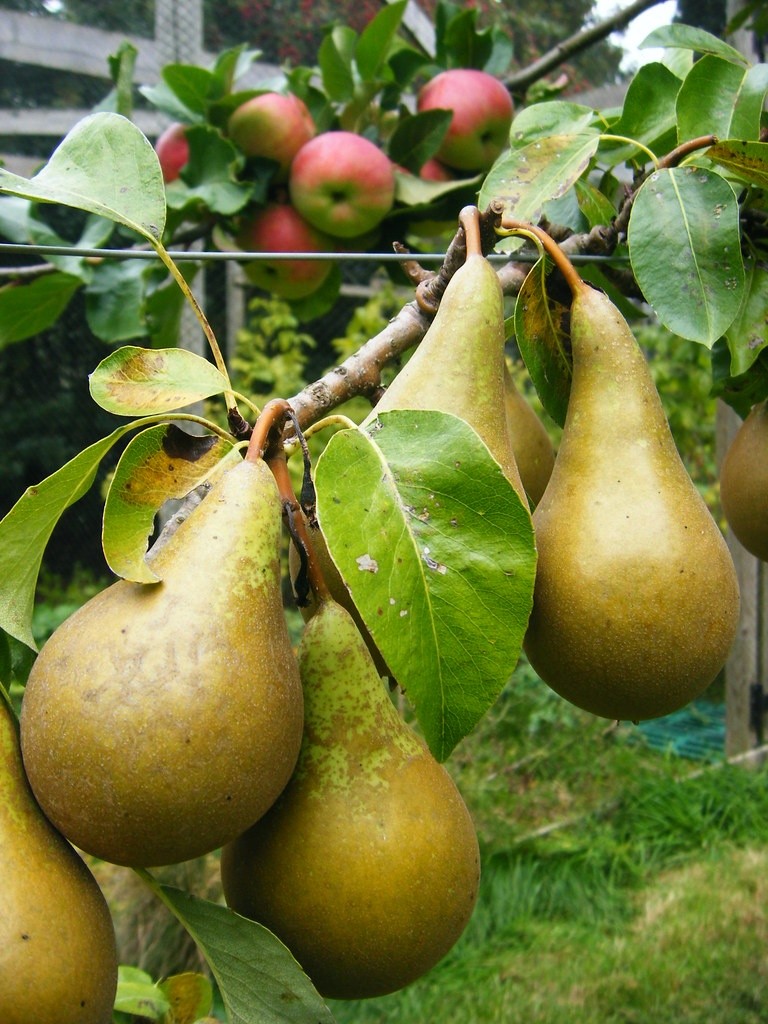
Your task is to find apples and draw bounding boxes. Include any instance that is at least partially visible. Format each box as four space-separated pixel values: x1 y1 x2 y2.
153 68 513 296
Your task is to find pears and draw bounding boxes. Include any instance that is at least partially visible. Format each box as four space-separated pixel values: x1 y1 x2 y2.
0 206 768 1024
